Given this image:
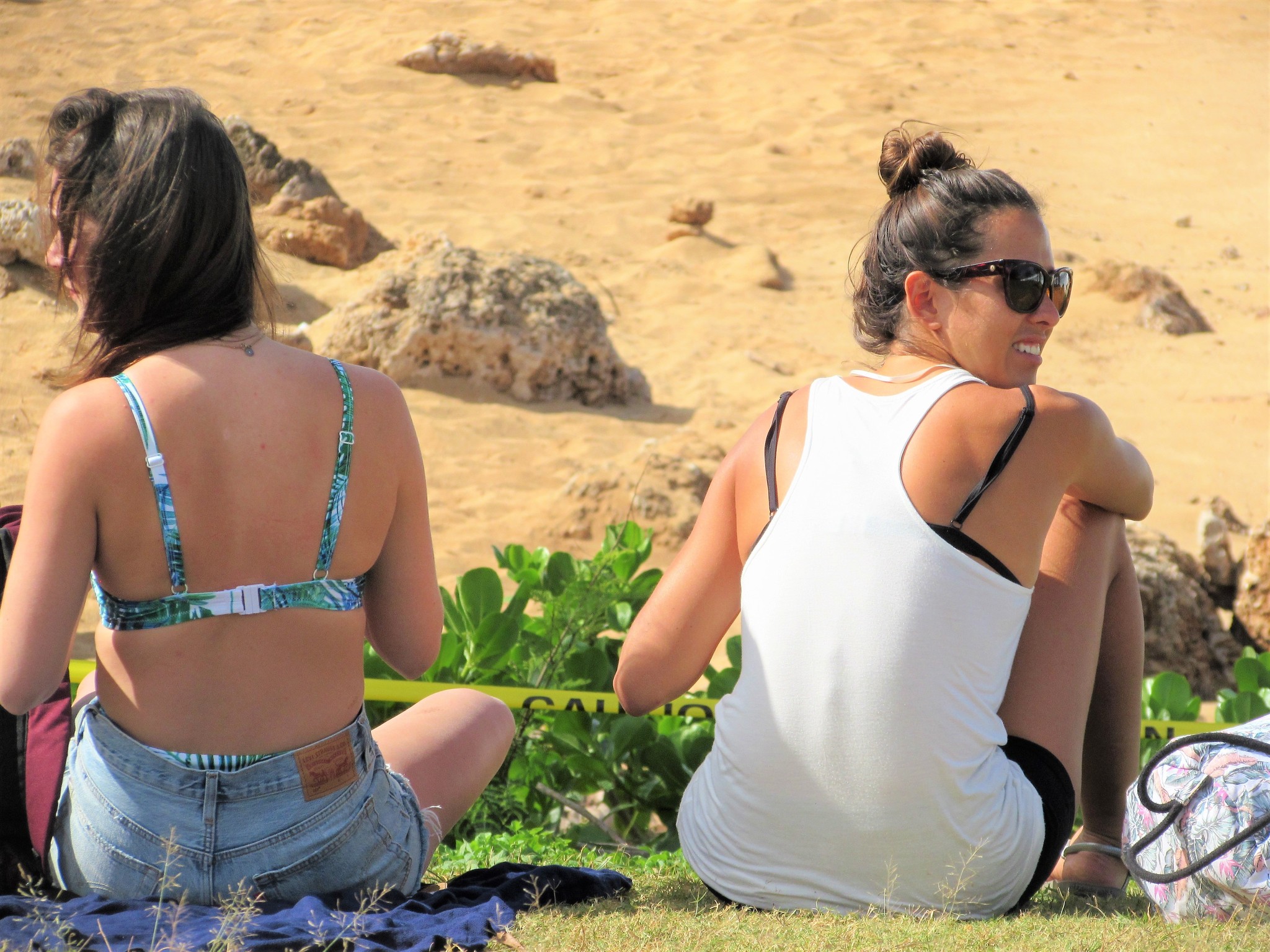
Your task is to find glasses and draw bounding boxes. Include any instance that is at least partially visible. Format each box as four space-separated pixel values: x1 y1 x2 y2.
932 259 1073 318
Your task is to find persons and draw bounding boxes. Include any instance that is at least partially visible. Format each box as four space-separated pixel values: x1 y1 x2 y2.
1 87 517 915
612 119 1156 920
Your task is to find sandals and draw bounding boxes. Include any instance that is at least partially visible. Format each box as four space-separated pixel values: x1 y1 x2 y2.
1040 843 1129 897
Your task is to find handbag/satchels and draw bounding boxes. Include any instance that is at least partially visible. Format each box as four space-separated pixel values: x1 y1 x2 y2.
1125 713 1270 925
